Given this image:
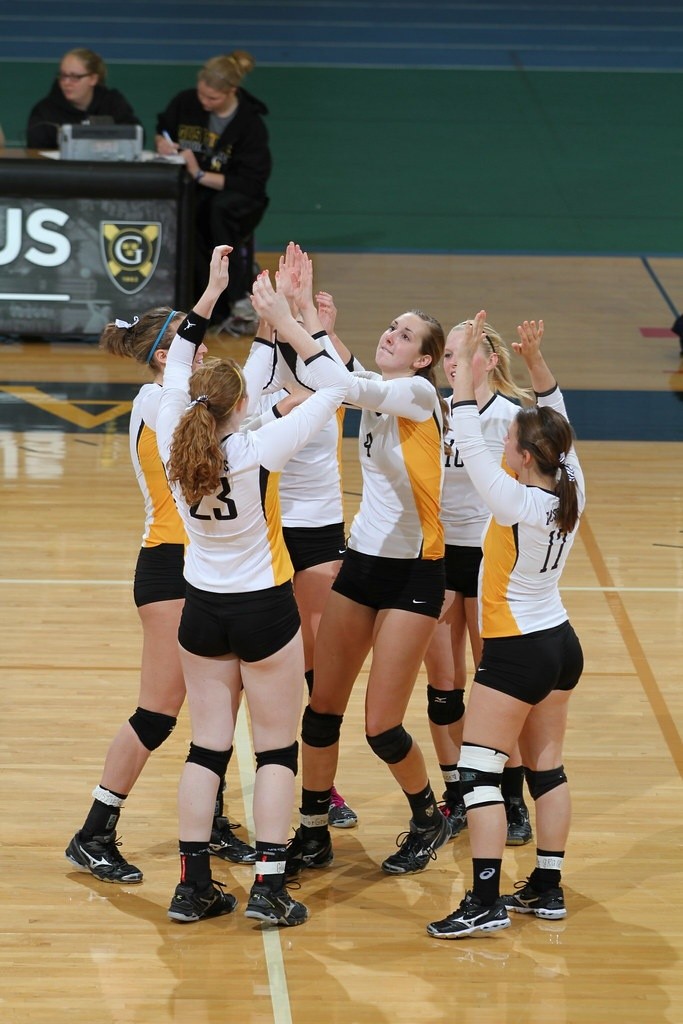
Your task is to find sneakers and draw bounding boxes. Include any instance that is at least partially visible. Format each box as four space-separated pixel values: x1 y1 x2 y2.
436 791 468 838
207 817 257 864
505 797 534 845
245 874 309 925
328 787 357 827
283 826 333 877
207 817 256 864
426 889 511 938
381 808 452 876
500 877 567 919
64 830 143 883
167 869 238 921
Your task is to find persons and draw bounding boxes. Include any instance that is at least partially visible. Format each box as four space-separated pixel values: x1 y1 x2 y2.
25 48 147 150
426 309 589 940
271 239 459 879
249 287 366 831
153 240 355 927
65 306 275 886
424 316 533 847
153 51 274 329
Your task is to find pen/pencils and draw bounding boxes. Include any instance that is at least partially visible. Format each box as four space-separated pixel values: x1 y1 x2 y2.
162 131 178 154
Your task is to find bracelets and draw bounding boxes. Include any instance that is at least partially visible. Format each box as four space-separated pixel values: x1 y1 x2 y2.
175 308 212 347
195 169 204 182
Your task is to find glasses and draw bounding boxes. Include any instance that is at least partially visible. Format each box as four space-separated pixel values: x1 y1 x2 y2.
57 73 92 82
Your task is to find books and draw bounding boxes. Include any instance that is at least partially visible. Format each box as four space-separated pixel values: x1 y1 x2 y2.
142 150 186 164
